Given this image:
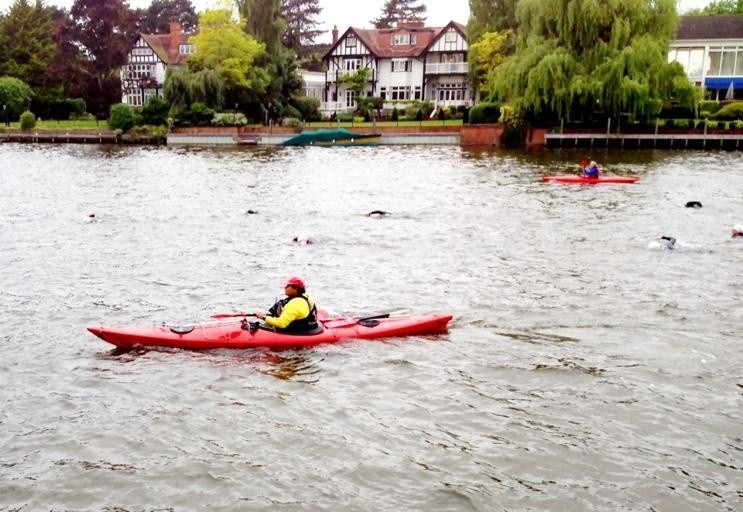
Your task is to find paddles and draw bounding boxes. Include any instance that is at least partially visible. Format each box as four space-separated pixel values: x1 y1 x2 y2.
211 313 271 317
320 308 410 328
581 160 587 178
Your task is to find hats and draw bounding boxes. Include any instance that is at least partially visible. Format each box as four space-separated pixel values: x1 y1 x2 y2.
281 277 305 288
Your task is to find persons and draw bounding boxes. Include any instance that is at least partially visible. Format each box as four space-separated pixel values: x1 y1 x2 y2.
583 160 598 180
255 277 317 334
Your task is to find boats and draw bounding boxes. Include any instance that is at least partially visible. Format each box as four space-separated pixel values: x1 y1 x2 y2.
87 308 452 351
540 173 640 184
